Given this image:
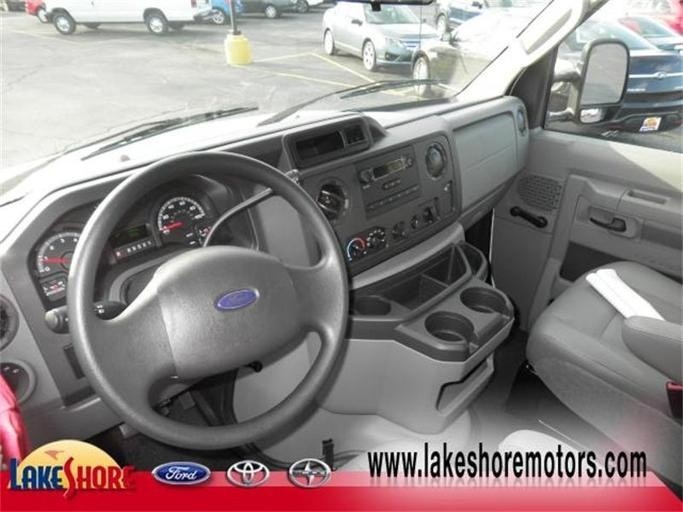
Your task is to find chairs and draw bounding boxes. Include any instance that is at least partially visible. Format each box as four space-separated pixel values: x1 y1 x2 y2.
524 257 683 488
